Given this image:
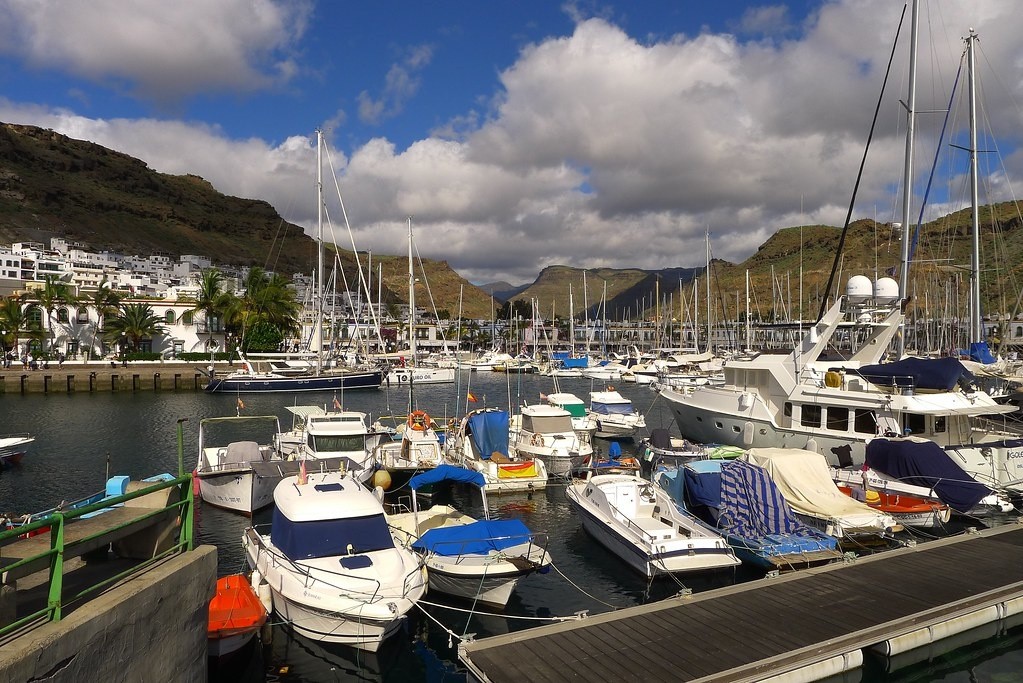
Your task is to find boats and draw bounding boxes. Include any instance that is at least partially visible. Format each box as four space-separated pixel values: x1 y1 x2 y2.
6 470 176 541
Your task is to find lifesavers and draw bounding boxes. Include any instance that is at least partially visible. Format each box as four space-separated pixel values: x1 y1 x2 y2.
408 410 431 431
825 372 841 387
531 433 544 447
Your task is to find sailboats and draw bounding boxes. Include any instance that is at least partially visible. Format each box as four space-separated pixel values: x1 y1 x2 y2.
194 0 1023 658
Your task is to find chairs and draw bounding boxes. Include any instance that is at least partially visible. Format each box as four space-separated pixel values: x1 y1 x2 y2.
223 441 264 469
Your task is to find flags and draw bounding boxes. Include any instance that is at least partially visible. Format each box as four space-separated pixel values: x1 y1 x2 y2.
468 391 478 404
237 399 244 409
334 398 343 408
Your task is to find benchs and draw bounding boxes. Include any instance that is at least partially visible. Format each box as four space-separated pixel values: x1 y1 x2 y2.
0 479 181 628
